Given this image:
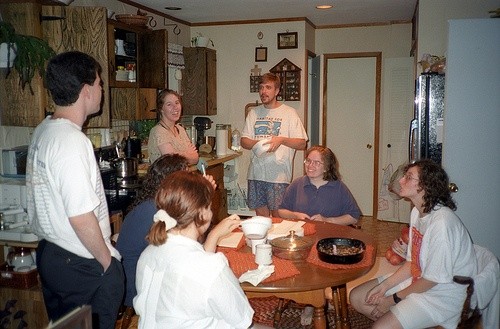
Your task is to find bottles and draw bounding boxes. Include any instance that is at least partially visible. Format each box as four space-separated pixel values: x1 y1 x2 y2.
231 129 240 151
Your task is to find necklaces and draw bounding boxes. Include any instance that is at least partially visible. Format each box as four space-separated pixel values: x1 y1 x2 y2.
161 119 178 138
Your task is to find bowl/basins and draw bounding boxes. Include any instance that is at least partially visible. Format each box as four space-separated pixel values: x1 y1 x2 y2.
241 222 269 239
252 138 271 157
115 14 149 26
269 230 315 260
316 237 366 265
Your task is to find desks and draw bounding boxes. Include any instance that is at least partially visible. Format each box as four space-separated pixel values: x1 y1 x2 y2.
215 217 377 329
138 151 243 224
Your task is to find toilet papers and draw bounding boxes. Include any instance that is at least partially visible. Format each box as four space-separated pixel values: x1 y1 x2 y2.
214 130 228 156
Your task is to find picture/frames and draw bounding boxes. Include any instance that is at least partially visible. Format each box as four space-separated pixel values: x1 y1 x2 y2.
255 47 267 62
277 32 298 50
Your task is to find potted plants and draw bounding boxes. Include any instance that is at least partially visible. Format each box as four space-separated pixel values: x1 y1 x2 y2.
191 32 215 47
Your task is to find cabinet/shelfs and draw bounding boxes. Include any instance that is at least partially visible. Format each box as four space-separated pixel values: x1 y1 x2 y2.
0 210 123 329
0 0 168 128
181 47 216 116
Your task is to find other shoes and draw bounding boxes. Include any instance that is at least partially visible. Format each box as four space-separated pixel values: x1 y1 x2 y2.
301 307 313 325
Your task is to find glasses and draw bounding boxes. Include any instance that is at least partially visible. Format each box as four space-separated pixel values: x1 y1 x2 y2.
304 158 324 168
403 175 419 183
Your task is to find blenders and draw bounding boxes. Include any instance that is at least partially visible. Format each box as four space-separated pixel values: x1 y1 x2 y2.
194 117 216 152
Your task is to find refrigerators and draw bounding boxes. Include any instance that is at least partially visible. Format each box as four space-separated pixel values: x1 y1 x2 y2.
408 72 445 169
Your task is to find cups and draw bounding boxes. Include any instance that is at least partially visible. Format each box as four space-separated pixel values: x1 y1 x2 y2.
251 239 266 254
255 244 272 264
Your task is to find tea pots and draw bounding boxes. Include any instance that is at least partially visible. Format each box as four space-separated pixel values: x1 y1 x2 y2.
7 247 36 272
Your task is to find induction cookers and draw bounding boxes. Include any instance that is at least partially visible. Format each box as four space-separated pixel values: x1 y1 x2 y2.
94 146 145 211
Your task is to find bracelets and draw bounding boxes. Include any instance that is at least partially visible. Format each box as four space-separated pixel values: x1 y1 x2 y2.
393 293 400 304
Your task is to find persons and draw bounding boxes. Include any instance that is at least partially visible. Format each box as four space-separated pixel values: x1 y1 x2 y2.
133 171 255 329
25 51 126 329
349 159 477 329
241 73 308 216
148 89 200 166
277 145 362 227
115 153 217 307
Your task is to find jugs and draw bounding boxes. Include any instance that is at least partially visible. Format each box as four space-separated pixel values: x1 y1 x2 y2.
186 125 197 145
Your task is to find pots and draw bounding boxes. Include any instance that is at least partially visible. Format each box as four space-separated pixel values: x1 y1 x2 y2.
113 157 138 178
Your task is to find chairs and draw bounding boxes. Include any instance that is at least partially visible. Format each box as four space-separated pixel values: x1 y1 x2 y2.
434 244 500 329
